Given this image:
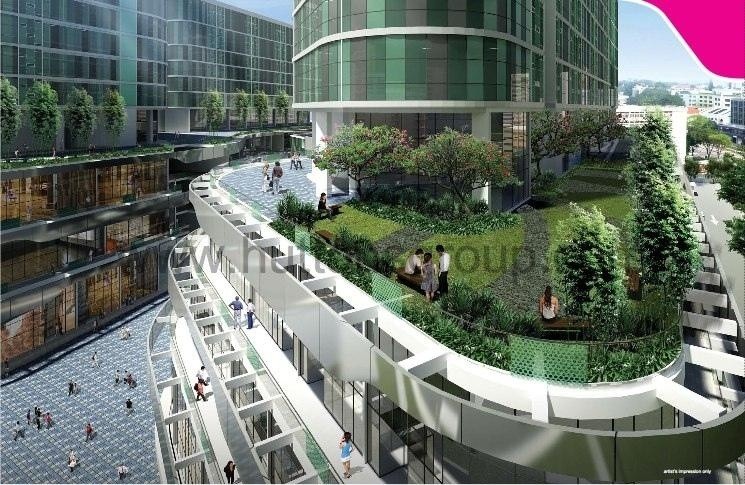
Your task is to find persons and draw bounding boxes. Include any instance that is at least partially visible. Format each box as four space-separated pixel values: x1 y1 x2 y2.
51 142 57 157
88 141 94 157
339 431 354 478
539 285 559 319
196 378 208 403
262 163 269 194
196 366 209 386
318 192 334 221
273 160 282 197
14 329 138 479
404 245 450 304
224 460 236 484
14 147 19 160
247 298 255 330
296 151 302 171
290 152 295 170
88 247 94 263
228 296 243 331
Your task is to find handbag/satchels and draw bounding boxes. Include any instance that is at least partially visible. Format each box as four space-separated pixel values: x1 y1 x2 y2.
194 383 198 390
269 181 273 187
